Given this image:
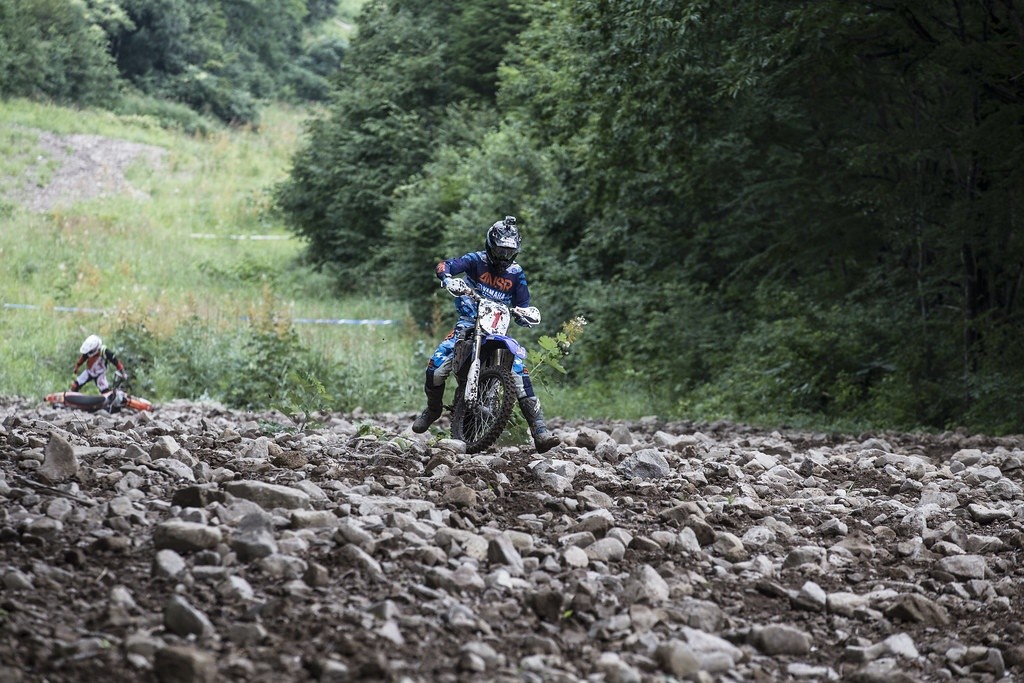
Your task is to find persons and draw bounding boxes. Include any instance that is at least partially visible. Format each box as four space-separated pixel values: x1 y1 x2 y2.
413 214 562 455
69 335 128 395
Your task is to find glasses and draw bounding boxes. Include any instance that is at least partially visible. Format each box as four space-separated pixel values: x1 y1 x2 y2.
493 246 515 260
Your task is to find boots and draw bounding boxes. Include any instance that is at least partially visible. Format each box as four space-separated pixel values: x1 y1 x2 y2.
412 381 445 433
519 395 560 454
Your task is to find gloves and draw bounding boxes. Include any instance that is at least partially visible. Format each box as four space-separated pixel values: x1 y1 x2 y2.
121 371 127 378
71 373 77 383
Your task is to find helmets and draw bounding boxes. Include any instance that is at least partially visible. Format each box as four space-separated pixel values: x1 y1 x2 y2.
485 216 522 272
80 335 102 358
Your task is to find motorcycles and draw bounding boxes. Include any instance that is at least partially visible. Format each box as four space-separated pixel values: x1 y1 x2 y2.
44 376 155 414
442 278 541 452
